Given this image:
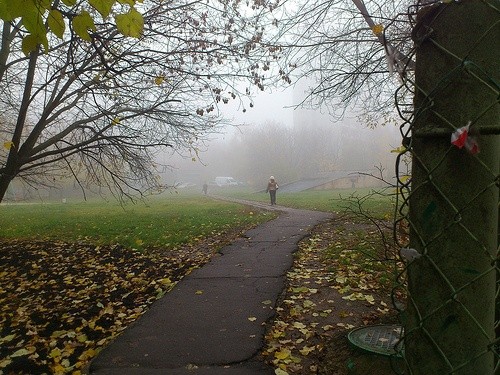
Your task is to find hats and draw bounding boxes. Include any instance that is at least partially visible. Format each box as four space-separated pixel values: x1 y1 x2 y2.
269 176 275 180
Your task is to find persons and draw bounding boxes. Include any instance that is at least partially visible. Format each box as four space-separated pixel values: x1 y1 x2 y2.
266 176 279 206
203 182 208 194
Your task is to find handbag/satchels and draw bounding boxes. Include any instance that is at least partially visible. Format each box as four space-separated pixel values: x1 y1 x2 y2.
275 183 279 190
